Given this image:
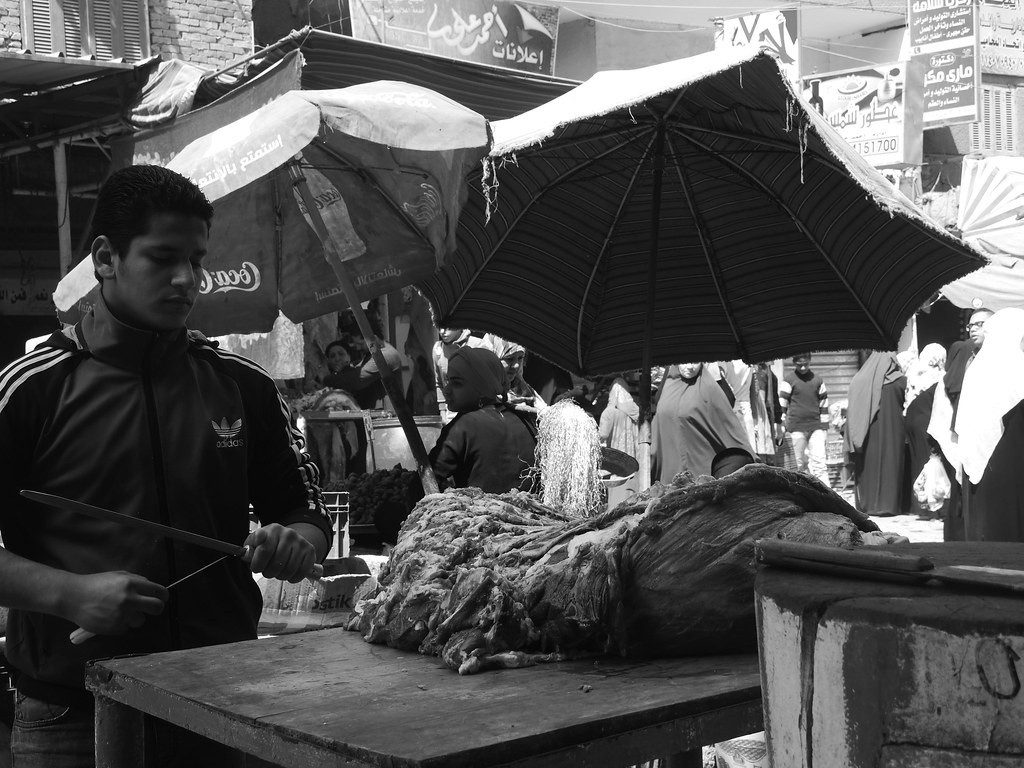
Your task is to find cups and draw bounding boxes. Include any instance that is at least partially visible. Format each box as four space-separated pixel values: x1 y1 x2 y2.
878 80 895 102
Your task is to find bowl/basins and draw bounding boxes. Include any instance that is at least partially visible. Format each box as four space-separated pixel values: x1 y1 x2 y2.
598 469 639 487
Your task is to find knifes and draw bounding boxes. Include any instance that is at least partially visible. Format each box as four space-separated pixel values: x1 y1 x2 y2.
20 490 323 580
757 539 1024 593
70 556 226 644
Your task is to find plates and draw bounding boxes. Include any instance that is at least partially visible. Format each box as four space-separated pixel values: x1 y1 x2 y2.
838 76 866 93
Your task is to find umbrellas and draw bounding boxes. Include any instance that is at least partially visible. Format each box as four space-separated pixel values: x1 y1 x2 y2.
411 42 992 496
52 80 492 497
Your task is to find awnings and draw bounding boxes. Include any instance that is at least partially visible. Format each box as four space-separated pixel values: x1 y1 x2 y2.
113 27 585 177
1 51 163 165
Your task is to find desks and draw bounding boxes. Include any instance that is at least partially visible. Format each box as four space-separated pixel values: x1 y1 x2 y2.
84 627 764 768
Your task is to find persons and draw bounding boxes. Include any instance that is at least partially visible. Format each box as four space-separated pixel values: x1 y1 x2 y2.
323 323 830 494
0 165 333 768
844 308 1024 542
